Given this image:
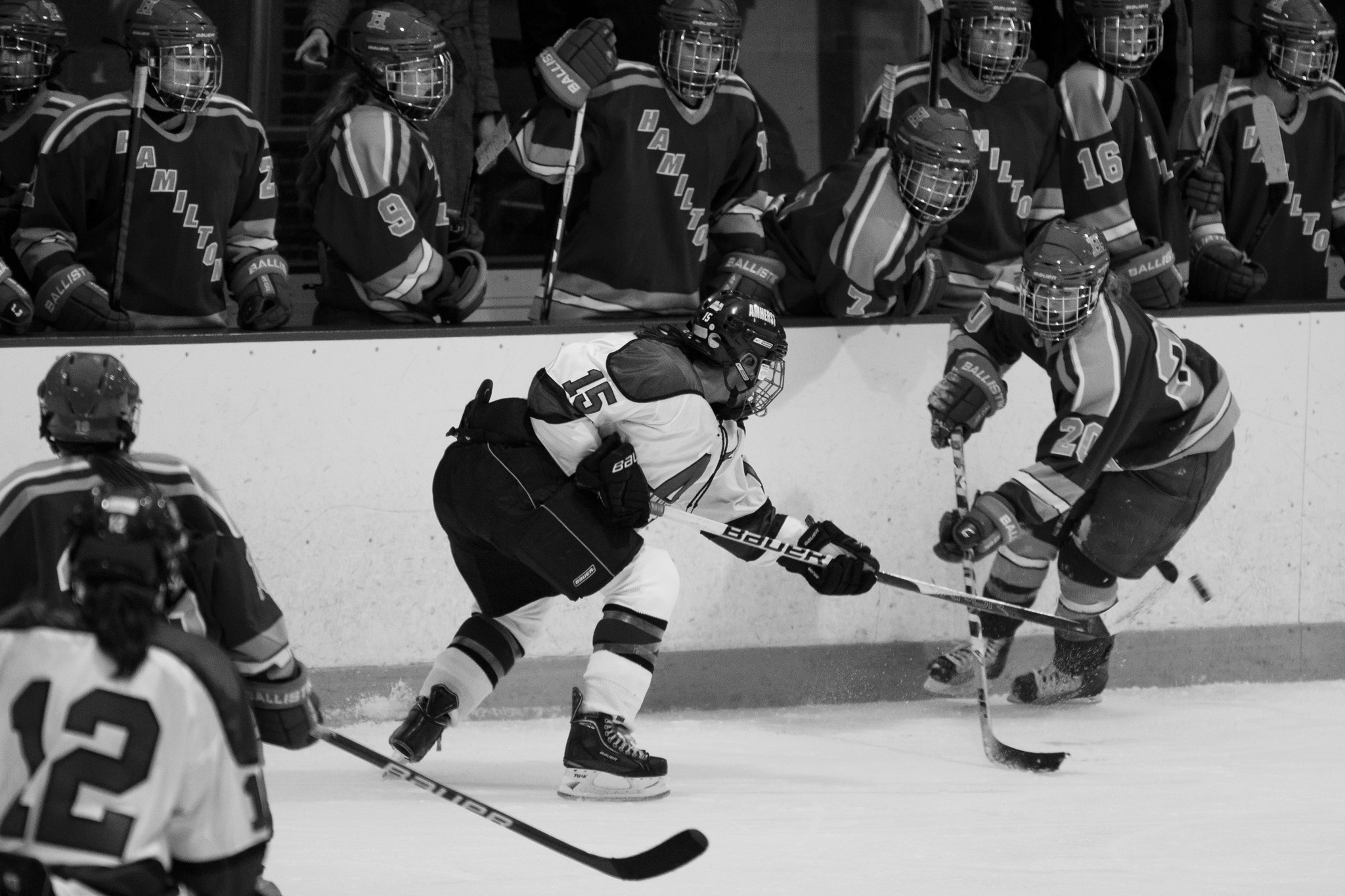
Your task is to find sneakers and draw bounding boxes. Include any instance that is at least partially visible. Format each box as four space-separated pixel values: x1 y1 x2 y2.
556 686 670 803
1008 654 1109 706
923 628 1013 696
384 685 457 781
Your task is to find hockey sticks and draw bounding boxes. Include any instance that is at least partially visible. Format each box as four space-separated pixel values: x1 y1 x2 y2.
1187 62 1235 238
533 94 588 322
647 497 1177 658
320 721 709 882
920 0 947 108
108 64 149 310
875 61 898 148
942 417 1071 775
456 111 515 249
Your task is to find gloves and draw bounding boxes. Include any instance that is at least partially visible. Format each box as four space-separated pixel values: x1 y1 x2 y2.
777 514 880 595
1192 223 1268 304
1 257 34 337
34 251 137 333
931 490 1021 563
1118 241 1183 311
927 352 1006 449
241 660 322 750
431 249 487 323
532 17 619 109
229 254 294 330
709 249 786 304
1174 149 1224 215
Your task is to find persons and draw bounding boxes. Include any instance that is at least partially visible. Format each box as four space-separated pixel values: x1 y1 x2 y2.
767 103 985 324
1053 0 1226 309
6 349 325 753
843 0 1068 317
501 0 789 323
6 0 297 334
0 0 97 335
1 471 289 895
384 288 884 803
923 215 1242 709
294 1 505 222
294 1 491 330
1173 1 1345 306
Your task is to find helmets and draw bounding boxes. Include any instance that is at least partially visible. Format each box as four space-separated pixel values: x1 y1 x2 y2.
890 104 981 226
124 0 224 112
1249 0 1340 88
0 0 67 106
36 351 139 445
1013 218 1112 341
948 0 1033 85
684 290 788 423
1074 0 1164 78
61 482 190 615
651 0 744 99
346 2 453 122
